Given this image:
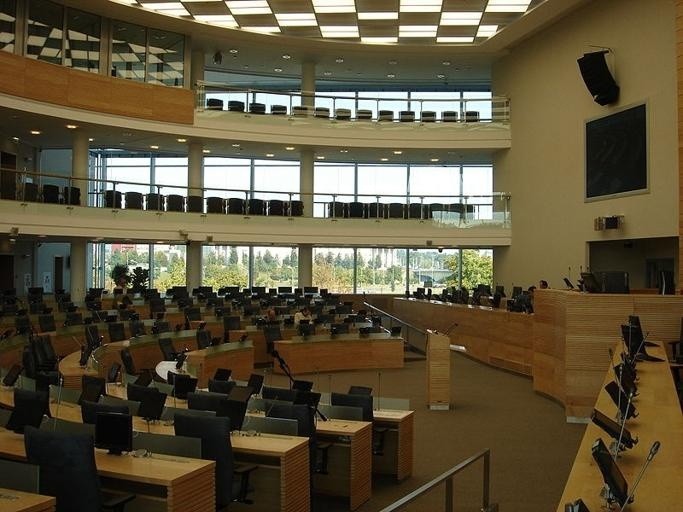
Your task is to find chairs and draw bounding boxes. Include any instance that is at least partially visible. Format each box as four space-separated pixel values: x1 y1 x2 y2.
328 201 474 219
19 183 81 206
103 190 305 219
207 99 479 122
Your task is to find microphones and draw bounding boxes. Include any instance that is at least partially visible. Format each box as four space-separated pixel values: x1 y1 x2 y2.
603 386 636 462
630 331 650 365
618 363 624 424
620 441 660 512
568 265 589 279
628 320 633 357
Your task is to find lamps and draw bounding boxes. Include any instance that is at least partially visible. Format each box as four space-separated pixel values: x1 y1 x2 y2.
213 52 222 64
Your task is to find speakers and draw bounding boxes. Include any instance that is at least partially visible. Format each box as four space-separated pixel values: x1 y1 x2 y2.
576 52 620 107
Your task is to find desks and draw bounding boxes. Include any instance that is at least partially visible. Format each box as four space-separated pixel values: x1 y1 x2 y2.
1 285 414 512
228 325 405 375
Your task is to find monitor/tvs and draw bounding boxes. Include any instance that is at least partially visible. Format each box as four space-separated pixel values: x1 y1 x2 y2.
170 376 198 400
77 383 103 405
563 271 675 295
227 385 255 402
292 379 314 393
136 390 168 420
620 315 645 360
605 380 636 420
613 362 637 397
293 391 321 411
3 285 522 388
215 398 248 433
590 408 633 449
94 412 133 456
591 438 628 508
4 399 46 434
565 499 590 512
348 385 373 395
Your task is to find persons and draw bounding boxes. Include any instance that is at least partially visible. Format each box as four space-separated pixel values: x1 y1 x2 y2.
269 306 275 319
540 280 548 288
112 275 137 321
293 307 312 329
